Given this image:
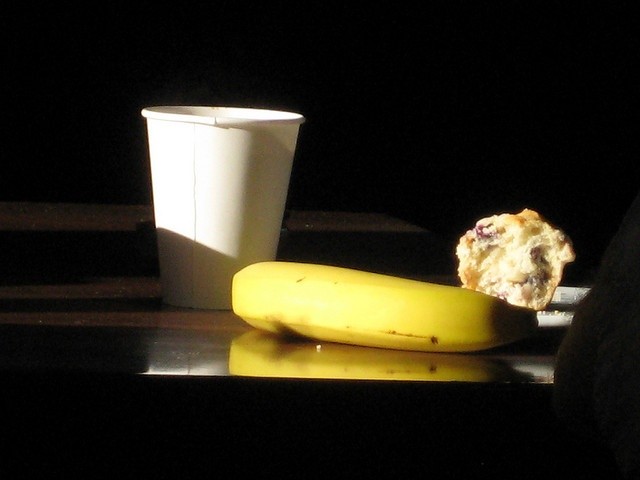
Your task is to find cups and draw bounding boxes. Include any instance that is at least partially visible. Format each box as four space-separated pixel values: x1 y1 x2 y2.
141 106 305 310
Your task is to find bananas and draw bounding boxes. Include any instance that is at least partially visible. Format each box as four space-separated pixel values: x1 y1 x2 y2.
227 330 511 382
232 259 539 354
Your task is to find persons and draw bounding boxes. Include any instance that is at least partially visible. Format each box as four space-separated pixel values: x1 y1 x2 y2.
552 204 640 480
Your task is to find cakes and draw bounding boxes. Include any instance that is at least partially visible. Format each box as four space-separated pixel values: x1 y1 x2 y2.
454 210 571 306
